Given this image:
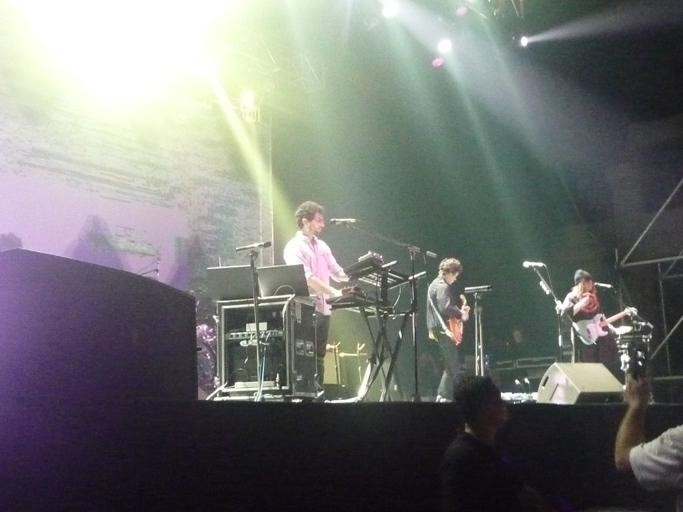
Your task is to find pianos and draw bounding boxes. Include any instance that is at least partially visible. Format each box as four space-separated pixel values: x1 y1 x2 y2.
326 294 391 318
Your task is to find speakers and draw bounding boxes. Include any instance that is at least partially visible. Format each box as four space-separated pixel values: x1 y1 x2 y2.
535 363 625 403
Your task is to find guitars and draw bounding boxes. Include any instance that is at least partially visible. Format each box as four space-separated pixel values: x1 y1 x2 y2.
576 307 637 346
449 295 470 344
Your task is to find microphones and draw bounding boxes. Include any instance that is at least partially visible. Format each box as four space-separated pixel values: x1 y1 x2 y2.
592 282 613 289
522 261 544 270
236 242 270 249
463 285 494 294
330 217 359 225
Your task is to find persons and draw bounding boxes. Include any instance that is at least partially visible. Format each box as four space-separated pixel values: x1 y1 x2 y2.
283 202 352 403
556 269 606 363
426 257 470 403
440 376 559 512
615 373 683 512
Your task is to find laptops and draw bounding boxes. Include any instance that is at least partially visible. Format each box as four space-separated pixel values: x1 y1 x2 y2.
256 266 316 298
206 266 256 297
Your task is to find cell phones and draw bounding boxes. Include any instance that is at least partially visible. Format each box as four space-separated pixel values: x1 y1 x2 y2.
632 343 647 380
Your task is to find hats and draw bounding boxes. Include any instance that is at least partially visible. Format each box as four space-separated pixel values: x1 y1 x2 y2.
574 268 593 284
